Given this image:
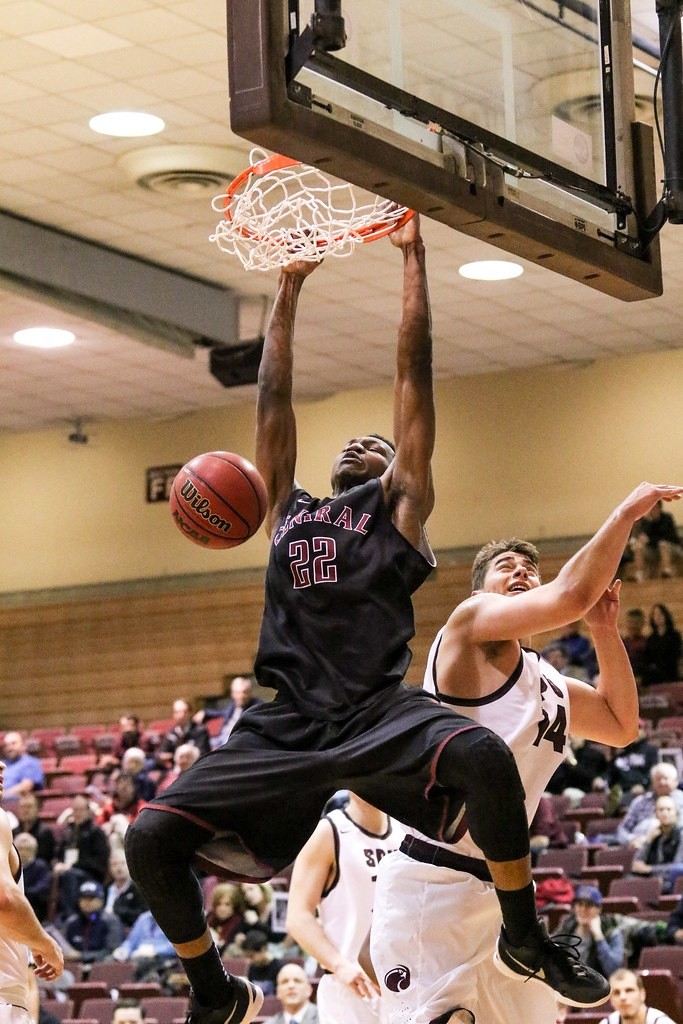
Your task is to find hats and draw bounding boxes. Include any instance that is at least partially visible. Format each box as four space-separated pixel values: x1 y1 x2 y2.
574 886 601 906
78 881 104 898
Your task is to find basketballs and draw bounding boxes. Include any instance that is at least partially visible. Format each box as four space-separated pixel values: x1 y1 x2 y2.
168 449 270 551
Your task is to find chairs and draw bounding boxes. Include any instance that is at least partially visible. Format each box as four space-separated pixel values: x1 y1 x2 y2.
0 680 683 1024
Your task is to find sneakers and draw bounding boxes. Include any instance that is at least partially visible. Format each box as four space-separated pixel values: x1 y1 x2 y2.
493 919 612 1009
183 972 265 1024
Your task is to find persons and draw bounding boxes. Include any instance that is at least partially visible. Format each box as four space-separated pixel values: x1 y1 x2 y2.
599 969 677 1023
0 759 64 1024
105 848 147 925
1 732 45 801
15 833 52 921
266 963 317 1024
123 215 613 1023
115 714 143 757
542 617 591 659
206 881 279 956
155 699 211 761
194 676 259 745
551 886 625 976
60 744 200 963
284 483 683 1024
12 794 58 862
